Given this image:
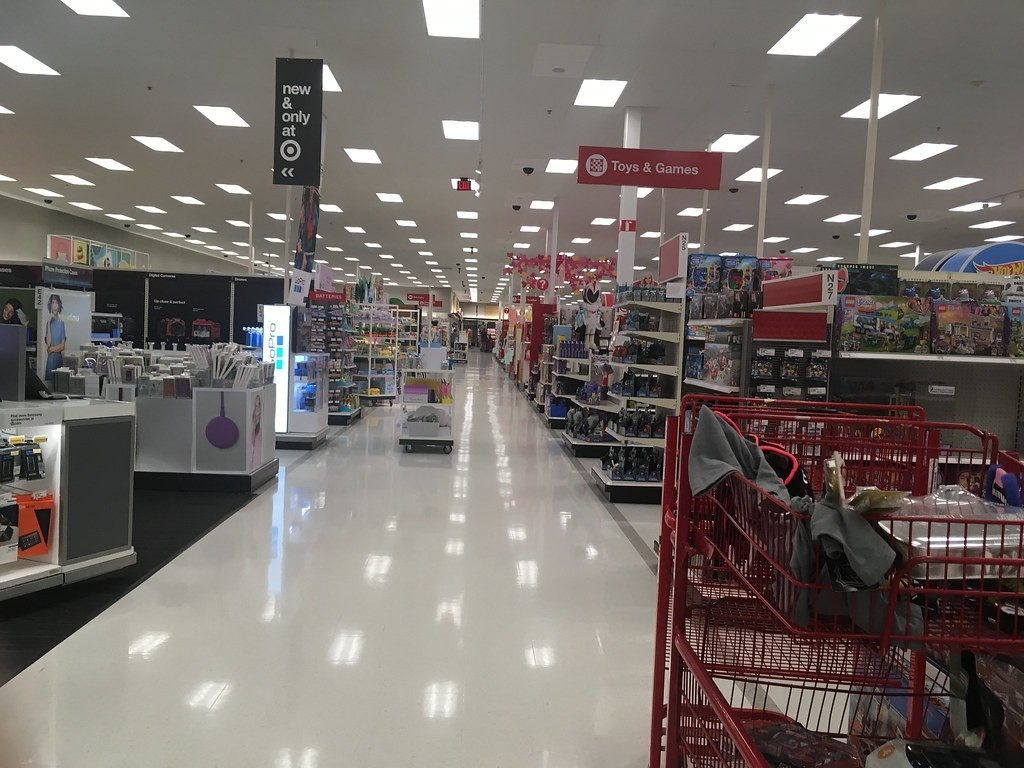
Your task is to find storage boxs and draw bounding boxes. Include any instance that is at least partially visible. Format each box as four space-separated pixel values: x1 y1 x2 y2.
704 344 740 387
689 294 703 318
747 292 763 318
932 300 1005 357
703 295 718 319
706 331 743 345
717 292 734 318
720 256 758 292
544 391 566 417
686 353 703 381
754 256 793 289
734 292 747 318
834 294 932 354
686 253 722 294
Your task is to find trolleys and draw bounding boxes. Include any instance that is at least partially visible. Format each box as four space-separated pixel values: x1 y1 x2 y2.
647 393 1024 767
449 330 469 363
398 346 455 454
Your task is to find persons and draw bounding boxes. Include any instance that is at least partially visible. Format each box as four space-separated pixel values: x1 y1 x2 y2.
640 273 654 286
294 186 318 273
44 295 66 378
0 299 29 326
248 395 263 470
581 283 602 350
479 321 488 351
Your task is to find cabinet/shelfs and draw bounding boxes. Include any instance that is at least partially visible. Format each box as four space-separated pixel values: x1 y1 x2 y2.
491 302 554 414
352 304 400 406
422 314 451 367
739 263 1024 509
562 280 622 458
591 232 793 505
310 301 361 426
387 308 420 395
543 305 591 431
